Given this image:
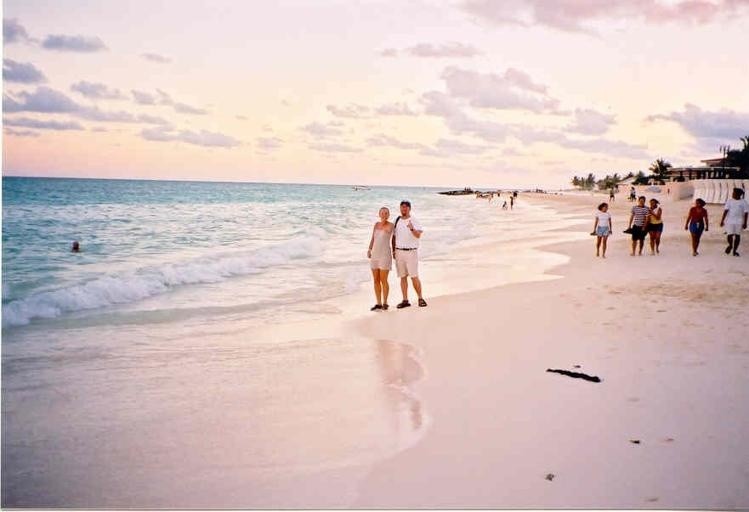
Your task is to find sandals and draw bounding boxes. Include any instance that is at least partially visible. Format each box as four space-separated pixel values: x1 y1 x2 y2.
418 298 428 307
396 299 412 308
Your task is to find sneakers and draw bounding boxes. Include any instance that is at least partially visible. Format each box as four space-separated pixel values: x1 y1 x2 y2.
380 303 389 311
623 228 634 234
370 304 382 311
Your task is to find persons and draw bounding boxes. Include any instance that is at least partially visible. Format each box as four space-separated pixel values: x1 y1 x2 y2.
475 185 636 210
392 202 427 308
628 196 651 256
721 189 748 256
645 199 663 255
367 208 394 311
685 199 708 256
594 203 612 258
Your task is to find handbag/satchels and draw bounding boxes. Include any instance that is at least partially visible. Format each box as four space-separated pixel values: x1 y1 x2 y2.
391 235 397 259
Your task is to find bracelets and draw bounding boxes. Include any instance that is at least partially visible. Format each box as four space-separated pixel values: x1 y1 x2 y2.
368 247 372 249
72 242 79 252
410 227 414 231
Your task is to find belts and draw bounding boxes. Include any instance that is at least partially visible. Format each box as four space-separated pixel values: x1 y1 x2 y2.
394 246 420 252
